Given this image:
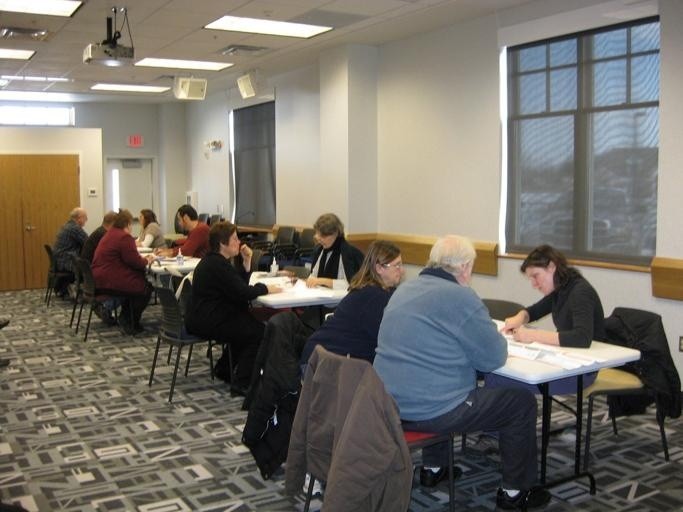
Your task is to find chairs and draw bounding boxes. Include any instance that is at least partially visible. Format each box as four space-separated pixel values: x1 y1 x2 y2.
43 243 74 307
148 287 234 402
67 252 119 327
266 310 308 467
482 299 527 323
167 278 224 376
551 307 669 462
303 344 457 512
73 259 135 341
251 225 320 274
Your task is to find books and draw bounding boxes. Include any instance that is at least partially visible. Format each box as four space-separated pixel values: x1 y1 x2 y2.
258 275 333 302
499 314 608 371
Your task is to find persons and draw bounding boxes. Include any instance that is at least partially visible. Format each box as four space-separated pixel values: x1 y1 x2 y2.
156 204 211 259
291 213 365 326
297 239 403 382
468 245 604 457
184 221 283 396
372 235 552 512
51 207 168 336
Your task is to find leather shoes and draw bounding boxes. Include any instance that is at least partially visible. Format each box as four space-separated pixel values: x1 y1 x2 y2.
494 488 551 511
420 466 463 488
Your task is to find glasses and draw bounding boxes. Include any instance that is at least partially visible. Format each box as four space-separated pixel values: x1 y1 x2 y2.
383 262 402 270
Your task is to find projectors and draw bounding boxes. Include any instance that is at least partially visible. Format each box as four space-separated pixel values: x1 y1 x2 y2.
82 43 134 69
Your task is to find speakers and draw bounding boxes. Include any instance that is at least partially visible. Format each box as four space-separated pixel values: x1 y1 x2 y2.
237 74 256 98
173 77 207 100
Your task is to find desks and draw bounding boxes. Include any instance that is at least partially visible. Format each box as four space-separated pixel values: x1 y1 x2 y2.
248 273 349 340
490 318 641 496
139 253 200 305
